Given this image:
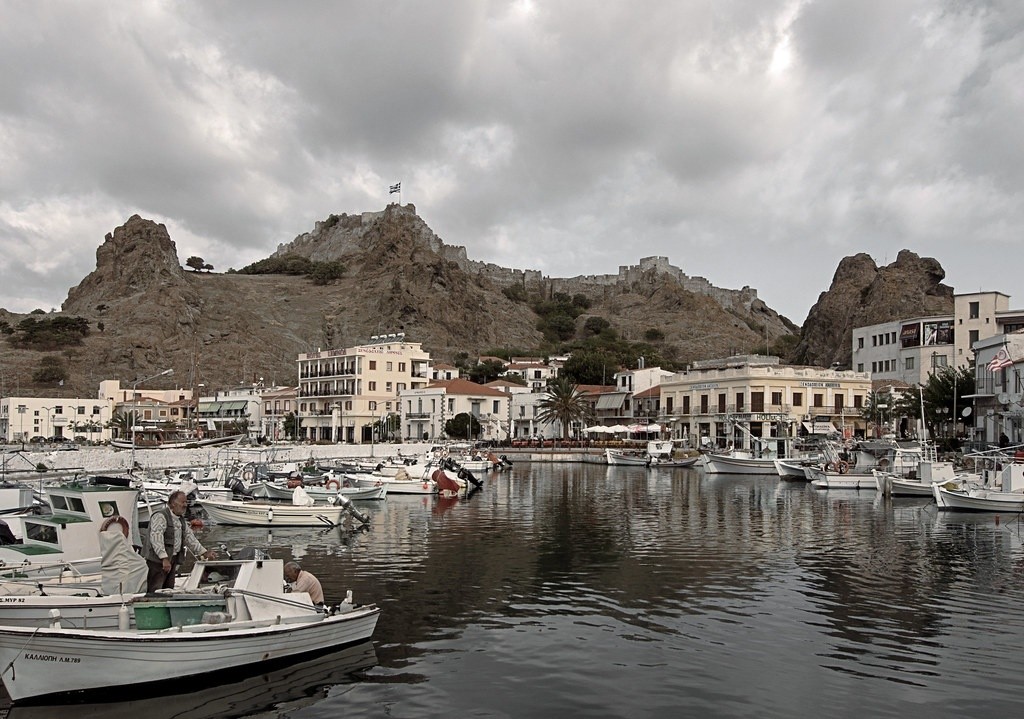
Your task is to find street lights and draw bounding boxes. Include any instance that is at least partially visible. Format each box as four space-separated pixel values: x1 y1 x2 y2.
553 410 558 448
469 412 472 441
371 398 396 457
68 405 81 440
859 384 891 439
519 413 522 437
94 405 108 446
42 406 56 442
132 369 174 468
580 411 585 448
15 407 29 435
271 386 302 446
936 364 975 438
645 409 650 440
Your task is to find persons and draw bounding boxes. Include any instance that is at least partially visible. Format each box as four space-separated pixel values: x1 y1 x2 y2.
283 561 325 613
140 490 218 593
838 448 848 463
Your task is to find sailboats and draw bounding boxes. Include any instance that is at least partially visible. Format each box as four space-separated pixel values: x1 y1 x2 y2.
109 338 246 450
871 387 984 497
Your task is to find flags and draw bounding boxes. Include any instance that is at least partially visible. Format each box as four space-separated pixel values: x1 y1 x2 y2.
389 183 400 195
985 345 1013 372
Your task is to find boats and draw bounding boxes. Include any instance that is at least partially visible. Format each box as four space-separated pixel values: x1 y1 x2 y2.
195 497 344 526
0 546 381 704
605 409 923 489
930 461 1024 511
345 474 440 494
0 441 503 596
264 482 389 499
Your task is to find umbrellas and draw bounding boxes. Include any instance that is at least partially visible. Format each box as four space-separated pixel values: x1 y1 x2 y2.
580 423 671 434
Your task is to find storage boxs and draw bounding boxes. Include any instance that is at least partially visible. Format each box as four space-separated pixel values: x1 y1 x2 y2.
166 600 226 628
133 601 171 630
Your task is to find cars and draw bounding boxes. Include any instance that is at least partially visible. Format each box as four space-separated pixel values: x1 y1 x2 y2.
31 436 70 442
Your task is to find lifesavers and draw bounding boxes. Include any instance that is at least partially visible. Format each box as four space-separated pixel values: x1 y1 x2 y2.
99 514 130 540
433 449 450 458
824 461 836 472
242 470 254 481
877 457 890 466
835 460 849 474
326 479 340 491
